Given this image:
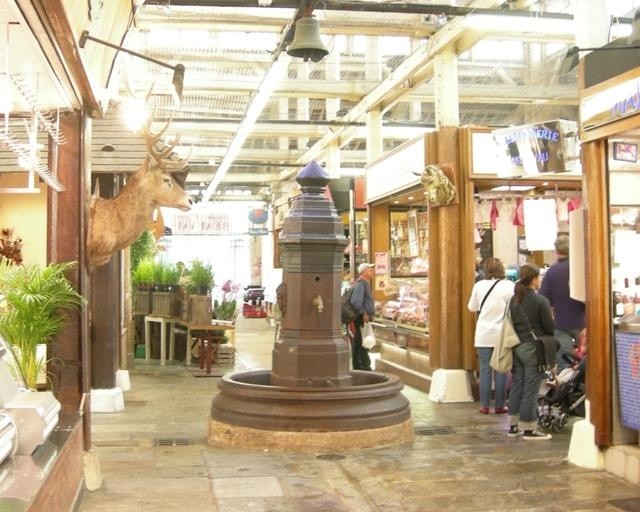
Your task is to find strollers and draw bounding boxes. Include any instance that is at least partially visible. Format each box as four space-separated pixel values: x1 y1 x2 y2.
537 338 586 434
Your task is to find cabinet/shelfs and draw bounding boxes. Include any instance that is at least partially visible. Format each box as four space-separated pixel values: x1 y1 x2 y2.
372 310 433 393
144 314 175 365
172 320 235 376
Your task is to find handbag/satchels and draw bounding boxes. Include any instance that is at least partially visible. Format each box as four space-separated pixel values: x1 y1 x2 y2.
488 294 521 375
536 334 557 366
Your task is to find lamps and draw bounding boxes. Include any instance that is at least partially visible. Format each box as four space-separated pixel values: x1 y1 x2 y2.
79 29 188 103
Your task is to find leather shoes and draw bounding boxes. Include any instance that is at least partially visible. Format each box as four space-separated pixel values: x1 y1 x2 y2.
480 406 489 414
495 406 509 414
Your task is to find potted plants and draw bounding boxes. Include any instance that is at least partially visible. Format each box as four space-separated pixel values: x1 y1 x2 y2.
131 258 214 322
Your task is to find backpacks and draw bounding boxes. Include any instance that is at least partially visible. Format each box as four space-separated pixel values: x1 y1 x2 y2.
341 280 366 325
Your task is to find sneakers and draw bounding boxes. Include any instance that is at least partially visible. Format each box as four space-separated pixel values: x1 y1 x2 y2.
507 426 523 437
520 430 553 441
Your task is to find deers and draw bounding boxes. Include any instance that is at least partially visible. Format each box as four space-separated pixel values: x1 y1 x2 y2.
86 108 193 268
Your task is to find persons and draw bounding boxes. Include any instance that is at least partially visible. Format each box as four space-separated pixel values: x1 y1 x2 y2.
176 262 190 276
534 233 587 419
342 262 376 373
465 257 515 415
548 335 586 388
222 279 232 296
508 262 555 441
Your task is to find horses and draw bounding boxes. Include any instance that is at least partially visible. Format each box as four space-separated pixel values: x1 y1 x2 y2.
411 166 456 209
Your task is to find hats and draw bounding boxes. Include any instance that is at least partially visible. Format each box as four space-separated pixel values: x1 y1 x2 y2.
358 263 377 274
